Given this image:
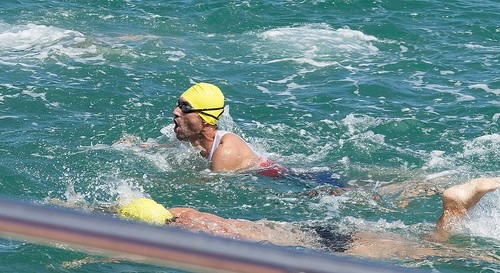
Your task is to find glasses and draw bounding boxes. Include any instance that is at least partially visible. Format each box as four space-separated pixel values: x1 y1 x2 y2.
177 102 225 120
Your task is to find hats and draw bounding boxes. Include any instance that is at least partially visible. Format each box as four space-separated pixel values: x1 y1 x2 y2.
181 82 224 125
116 198 173 226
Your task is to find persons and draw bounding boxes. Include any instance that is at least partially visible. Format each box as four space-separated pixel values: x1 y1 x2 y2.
58 176 500 270
133 81 457 212
